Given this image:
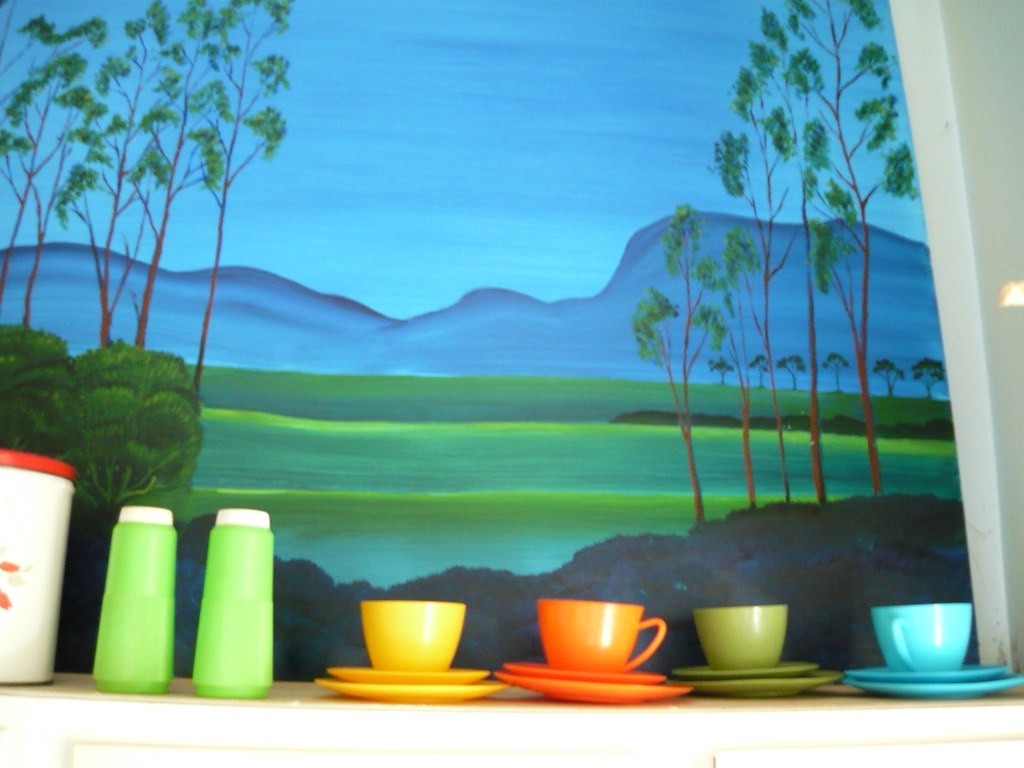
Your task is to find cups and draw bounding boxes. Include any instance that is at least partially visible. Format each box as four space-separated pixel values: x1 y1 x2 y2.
870 603 974 671
359 599 467 676
694 603 790 664
537 598 666 676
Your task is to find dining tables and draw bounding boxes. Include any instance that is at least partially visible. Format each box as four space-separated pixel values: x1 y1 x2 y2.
1 670 1024 709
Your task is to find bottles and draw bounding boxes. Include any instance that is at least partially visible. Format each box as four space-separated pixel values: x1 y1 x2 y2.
93 503 179 695
190 508 279 698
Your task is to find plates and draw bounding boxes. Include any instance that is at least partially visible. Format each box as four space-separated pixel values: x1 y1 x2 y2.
842 673 1023 701
669 660 819 680
310 679 516 702
840 660 1014 682
498 674 696 707
675 671 845 700
328 664 494 683
501 660 666 684
